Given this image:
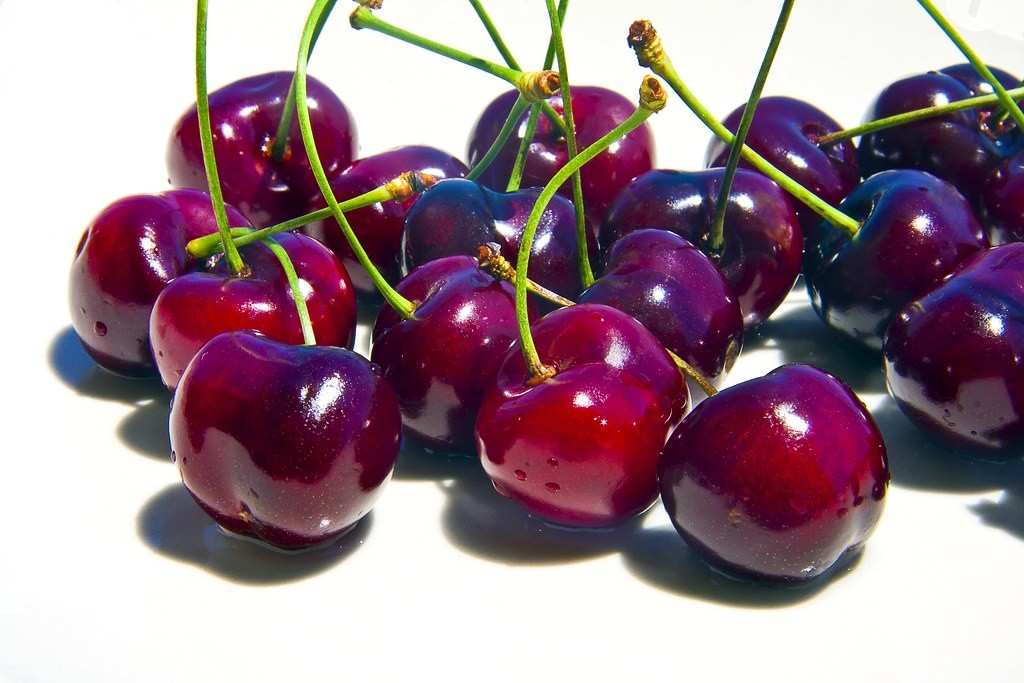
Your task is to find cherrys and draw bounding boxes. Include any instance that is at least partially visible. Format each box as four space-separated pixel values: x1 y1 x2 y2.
69 0 1024 583
